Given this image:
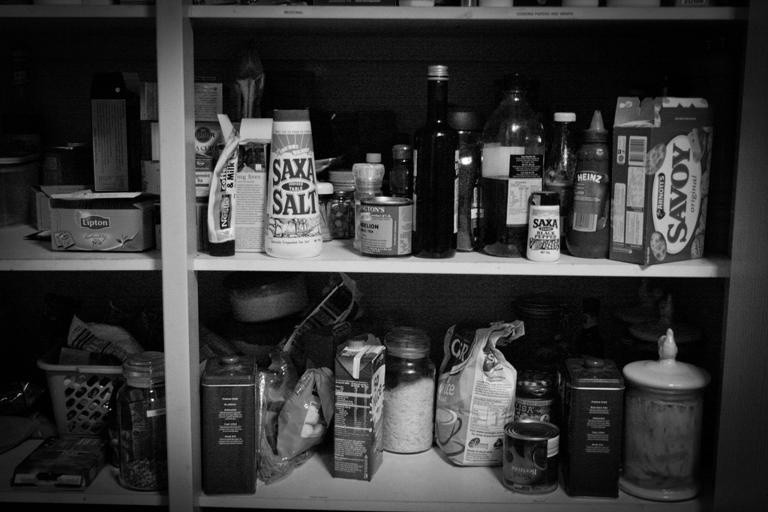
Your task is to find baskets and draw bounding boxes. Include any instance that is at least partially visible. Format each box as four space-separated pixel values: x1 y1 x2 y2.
37 361 123 442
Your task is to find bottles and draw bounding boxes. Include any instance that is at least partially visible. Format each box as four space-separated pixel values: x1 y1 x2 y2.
386 145 412 196
263 109 323 261
327 168 356 240
411 63 459 259
114 352 168 492
544 110 575 251
382 325 436 456
478 71 545 259
351 162 386 252
449 109 477 252
565 109 611 260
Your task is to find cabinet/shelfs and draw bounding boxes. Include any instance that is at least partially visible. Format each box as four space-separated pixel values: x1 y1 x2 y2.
178 0 768 512
0 1 178 511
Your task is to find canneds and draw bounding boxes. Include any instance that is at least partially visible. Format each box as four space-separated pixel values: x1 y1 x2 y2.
0 154 40 233
41 141 86 186
222 272 311 325
359 195 414 257
501 418 562 495
515 367 554 425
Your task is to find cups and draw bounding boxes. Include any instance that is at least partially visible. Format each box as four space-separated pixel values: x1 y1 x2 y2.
526 191 560 263
